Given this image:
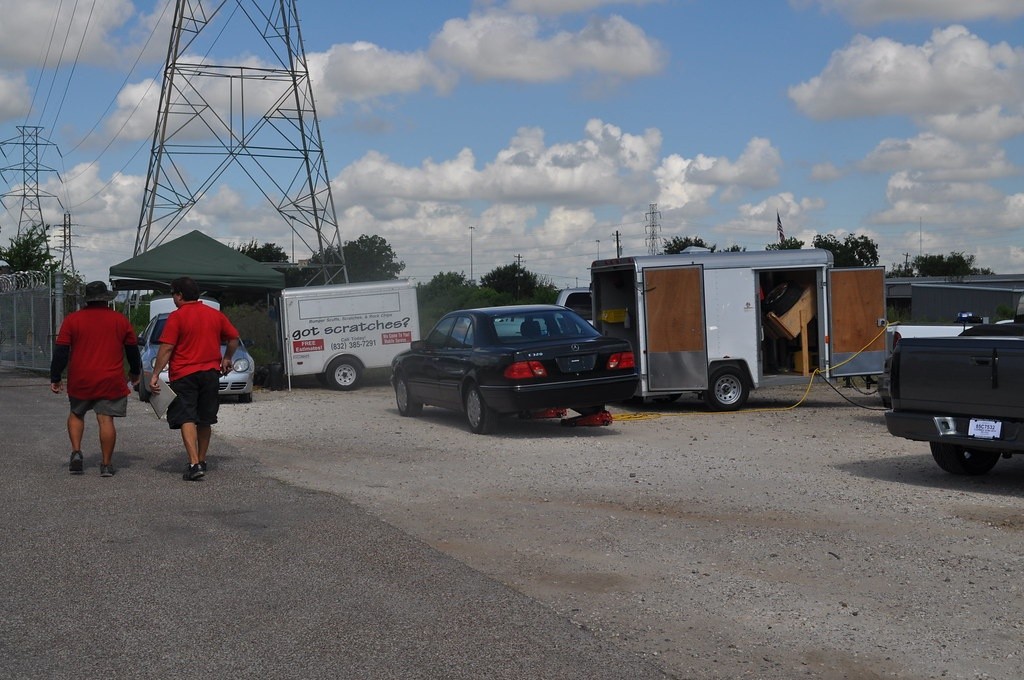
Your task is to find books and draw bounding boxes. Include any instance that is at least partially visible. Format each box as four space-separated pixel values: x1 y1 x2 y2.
148 378 176 419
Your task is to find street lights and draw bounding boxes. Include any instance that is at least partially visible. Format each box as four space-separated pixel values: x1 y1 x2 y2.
467 225 476 286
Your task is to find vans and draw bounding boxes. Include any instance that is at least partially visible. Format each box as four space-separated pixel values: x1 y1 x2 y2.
556 288 595 329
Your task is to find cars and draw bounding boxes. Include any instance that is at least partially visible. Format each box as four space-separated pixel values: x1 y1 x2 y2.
391 303 641 427
135 311 255 402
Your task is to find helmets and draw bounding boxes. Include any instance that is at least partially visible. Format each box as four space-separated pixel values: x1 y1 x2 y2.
83 281 119 302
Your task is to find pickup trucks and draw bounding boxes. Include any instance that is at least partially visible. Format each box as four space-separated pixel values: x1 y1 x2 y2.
883 287 1023 479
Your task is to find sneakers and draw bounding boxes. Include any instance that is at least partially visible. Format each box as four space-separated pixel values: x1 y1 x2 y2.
99 464 113 477
69 450 83 473
183 461 206 480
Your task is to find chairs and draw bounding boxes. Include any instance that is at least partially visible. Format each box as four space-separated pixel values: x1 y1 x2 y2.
519 320 547 341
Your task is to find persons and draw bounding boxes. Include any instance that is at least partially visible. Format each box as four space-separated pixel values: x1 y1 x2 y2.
148 277 240 481
49 281 141 476
